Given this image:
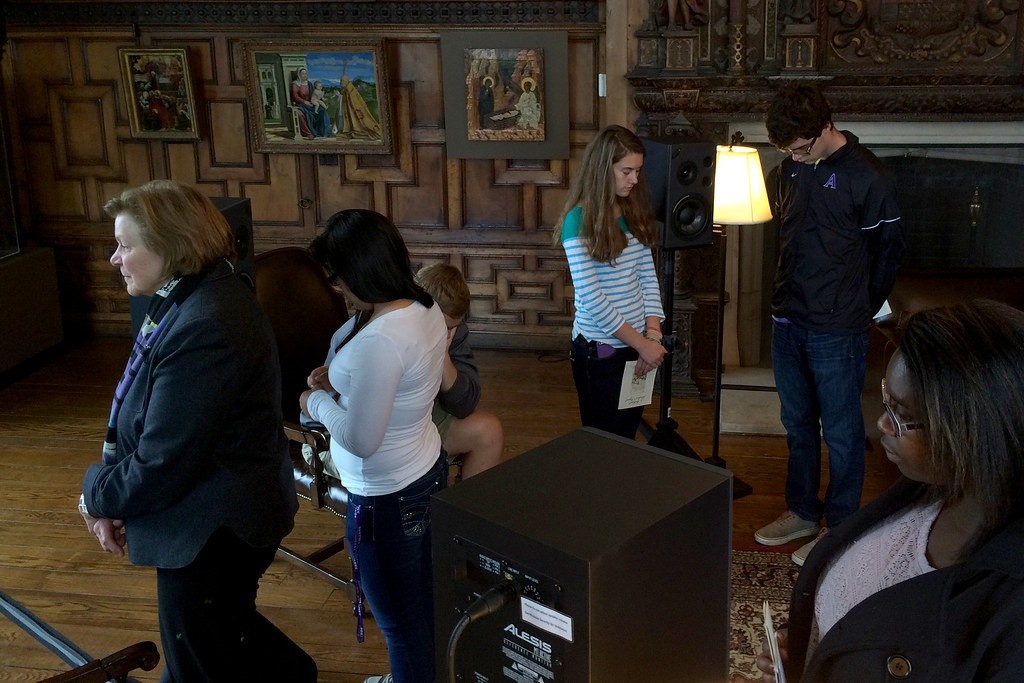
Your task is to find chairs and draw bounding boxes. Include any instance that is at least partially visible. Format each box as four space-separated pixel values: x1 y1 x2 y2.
239 245 462 601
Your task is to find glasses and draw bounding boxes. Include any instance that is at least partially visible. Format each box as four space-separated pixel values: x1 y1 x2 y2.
881 377 930 439
780 123 825 156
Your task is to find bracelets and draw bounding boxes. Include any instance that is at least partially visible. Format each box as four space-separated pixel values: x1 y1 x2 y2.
645 326 663 344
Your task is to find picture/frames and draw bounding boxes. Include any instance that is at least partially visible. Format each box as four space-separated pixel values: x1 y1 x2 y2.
439 29 571 160
115 44 201 144
239 36 397 157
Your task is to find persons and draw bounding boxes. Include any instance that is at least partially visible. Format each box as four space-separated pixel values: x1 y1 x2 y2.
560 124 669 442
304 262 503 590
79 179 321 683
755 299 1023 683
753 80 907 565
297 208 449 682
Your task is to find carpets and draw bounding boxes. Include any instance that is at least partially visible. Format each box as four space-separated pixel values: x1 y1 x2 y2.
729 549 801 683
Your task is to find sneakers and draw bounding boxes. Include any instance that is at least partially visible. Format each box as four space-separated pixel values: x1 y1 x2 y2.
754 511 820 546
791 527 831 566
364 674 393 683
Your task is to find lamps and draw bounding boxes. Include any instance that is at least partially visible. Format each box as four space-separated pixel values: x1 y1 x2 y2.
703 144 772 499
